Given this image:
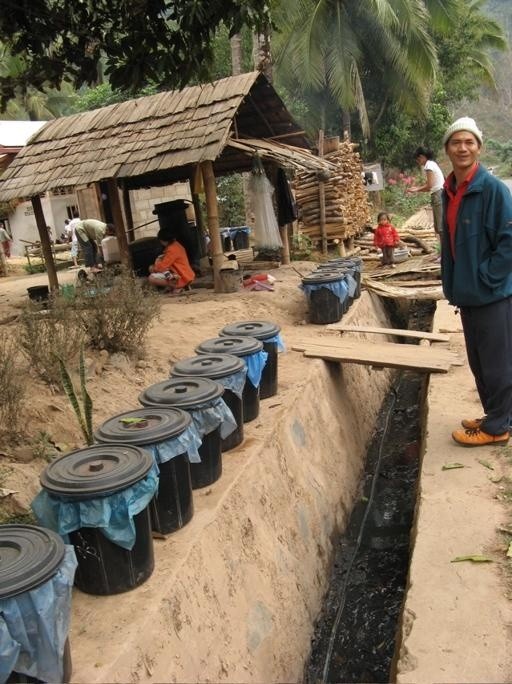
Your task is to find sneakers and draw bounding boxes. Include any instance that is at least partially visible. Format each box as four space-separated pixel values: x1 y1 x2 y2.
452 428 510 446
462 417 488 428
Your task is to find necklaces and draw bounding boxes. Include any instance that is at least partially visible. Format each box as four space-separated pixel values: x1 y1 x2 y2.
410 145 444 243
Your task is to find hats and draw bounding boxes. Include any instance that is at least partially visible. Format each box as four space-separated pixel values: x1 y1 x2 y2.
443 118 483 148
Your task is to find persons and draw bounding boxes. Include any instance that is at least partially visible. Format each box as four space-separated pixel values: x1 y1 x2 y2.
428 111 512 447
66 210 82 270
72 219 114 273
151 245 182 282
374 212 400 269
63 218 72 238
148 226 195 296
47 225 54 246
0 223 13 259
